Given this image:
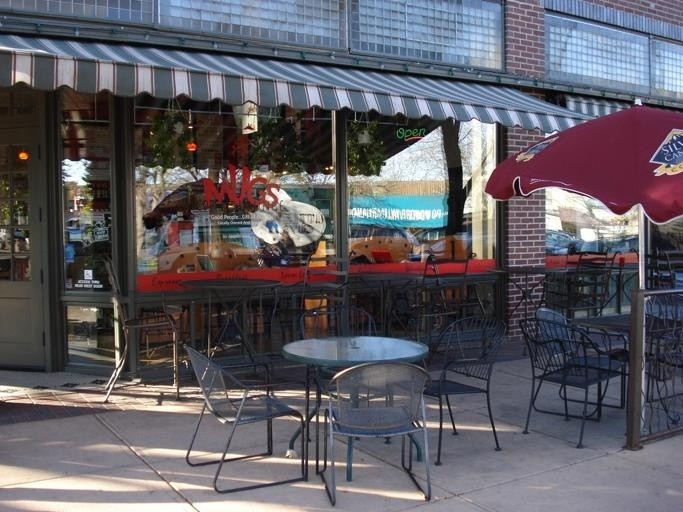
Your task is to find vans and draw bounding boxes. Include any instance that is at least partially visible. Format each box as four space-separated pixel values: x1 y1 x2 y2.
348 222 421 262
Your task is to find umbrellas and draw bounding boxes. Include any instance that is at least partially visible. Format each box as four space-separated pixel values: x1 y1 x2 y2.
484 98 683 433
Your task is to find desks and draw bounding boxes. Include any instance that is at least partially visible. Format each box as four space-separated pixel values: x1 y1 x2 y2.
573 307 680 435
282 334 432 481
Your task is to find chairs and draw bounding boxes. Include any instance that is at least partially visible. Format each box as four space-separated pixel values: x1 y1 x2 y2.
100 247 680 403
179 342 306 494
517 316 632 452
383 317 509 466
321 360 433 504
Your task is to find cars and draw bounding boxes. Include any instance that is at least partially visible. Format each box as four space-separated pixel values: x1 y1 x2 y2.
408 234 473 261
545 231 637 256
